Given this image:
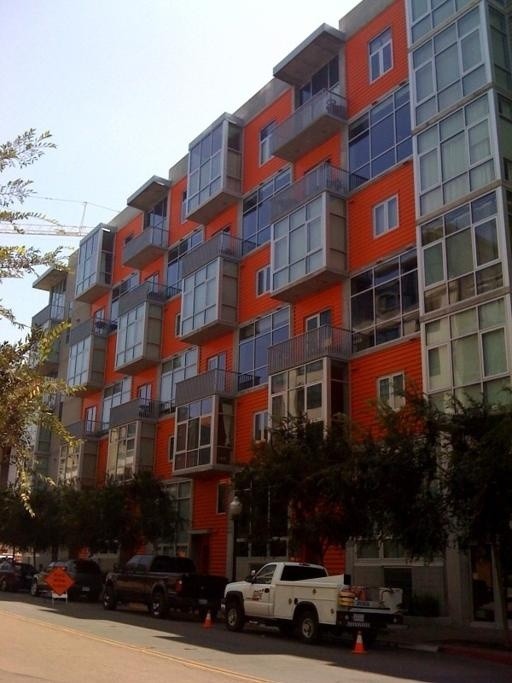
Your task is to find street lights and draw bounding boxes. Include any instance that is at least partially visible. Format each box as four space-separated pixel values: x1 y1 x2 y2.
228 495 243 581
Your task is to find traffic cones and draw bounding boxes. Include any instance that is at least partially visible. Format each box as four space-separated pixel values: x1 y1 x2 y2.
201 608 217 627
359 588 365 601
352 629 367 653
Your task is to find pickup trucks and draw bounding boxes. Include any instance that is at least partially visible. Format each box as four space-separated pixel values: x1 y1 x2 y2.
222 560 410 645
101 552 229 617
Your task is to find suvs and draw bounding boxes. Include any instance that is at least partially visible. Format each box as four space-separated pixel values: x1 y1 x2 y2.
31 556 103 603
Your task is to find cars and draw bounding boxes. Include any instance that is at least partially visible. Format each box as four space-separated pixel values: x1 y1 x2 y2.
1 558 38 592
0 555 16 563
